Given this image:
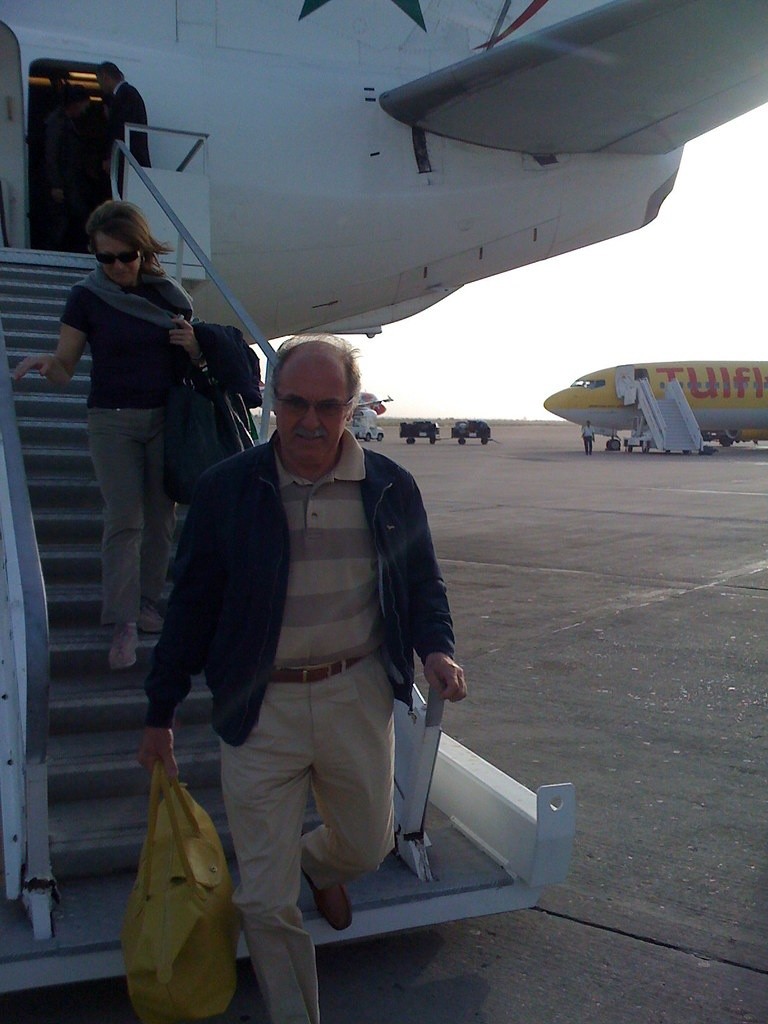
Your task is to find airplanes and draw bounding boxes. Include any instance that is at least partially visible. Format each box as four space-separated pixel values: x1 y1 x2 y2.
544 359 768 456
1 0 768 993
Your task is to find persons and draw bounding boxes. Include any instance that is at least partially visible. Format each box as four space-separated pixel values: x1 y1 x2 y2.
142 339 469 1024
581 420 595 455
34 61 152 252
12 200 210 669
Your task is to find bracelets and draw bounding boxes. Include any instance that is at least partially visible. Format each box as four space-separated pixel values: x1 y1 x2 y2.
191 351 205 360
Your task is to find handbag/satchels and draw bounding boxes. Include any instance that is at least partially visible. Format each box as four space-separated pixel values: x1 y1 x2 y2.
120 761 240 1024
163 382 224 504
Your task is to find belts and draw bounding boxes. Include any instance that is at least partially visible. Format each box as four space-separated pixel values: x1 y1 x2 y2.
271 650 373 683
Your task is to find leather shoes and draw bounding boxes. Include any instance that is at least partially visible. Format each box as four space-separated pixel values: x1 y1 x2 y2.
301 832 352 931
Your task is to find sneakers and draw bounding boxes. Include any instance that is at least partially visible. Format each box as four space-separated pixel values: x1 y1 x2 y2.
137 598 164 633
108 622 138 670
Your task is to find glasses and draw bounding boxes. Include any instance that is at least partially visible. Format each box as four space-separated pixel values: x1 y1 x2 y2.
273 387 354 416
92 238 138 264
